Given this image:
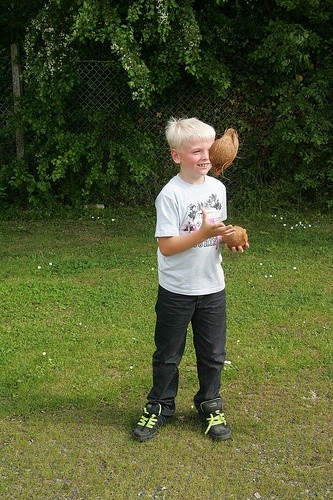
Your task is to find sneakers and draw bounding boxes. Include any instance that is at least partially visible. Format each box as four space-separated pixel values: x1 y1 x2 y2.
194 398 231 441
132 402 177 441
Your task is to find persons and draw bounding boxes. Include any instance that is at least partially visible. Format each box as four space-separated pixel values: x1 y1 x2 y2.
131 116 251 441
